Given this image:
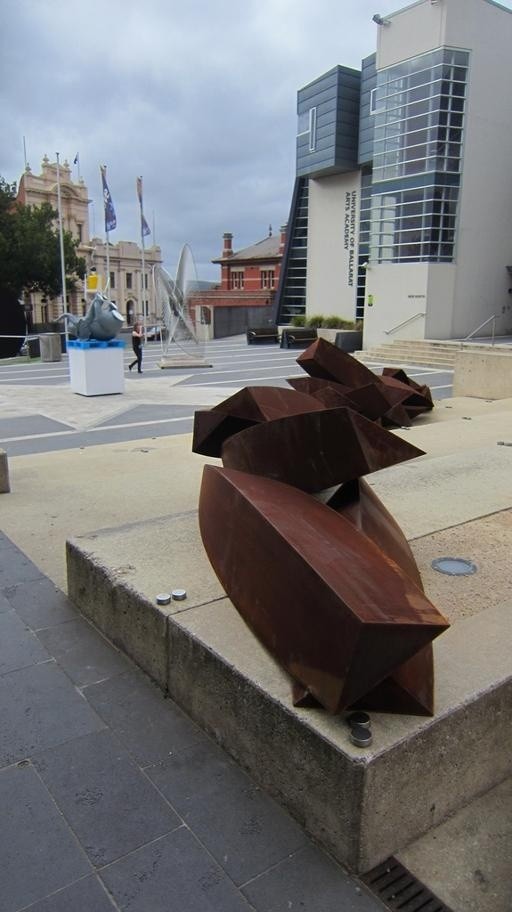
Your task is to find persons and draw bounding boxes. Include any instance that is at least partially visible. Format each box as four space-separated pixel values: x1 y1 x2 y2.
129 322 143 374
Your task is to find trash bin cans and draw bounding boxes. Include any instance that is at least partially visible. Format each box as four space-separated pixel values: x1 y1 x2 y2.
39 333 62 362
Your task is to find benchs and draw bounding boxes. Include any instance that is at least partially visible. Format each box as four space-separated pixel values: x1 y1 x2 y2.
246 327 279 345
279 327 318 349
